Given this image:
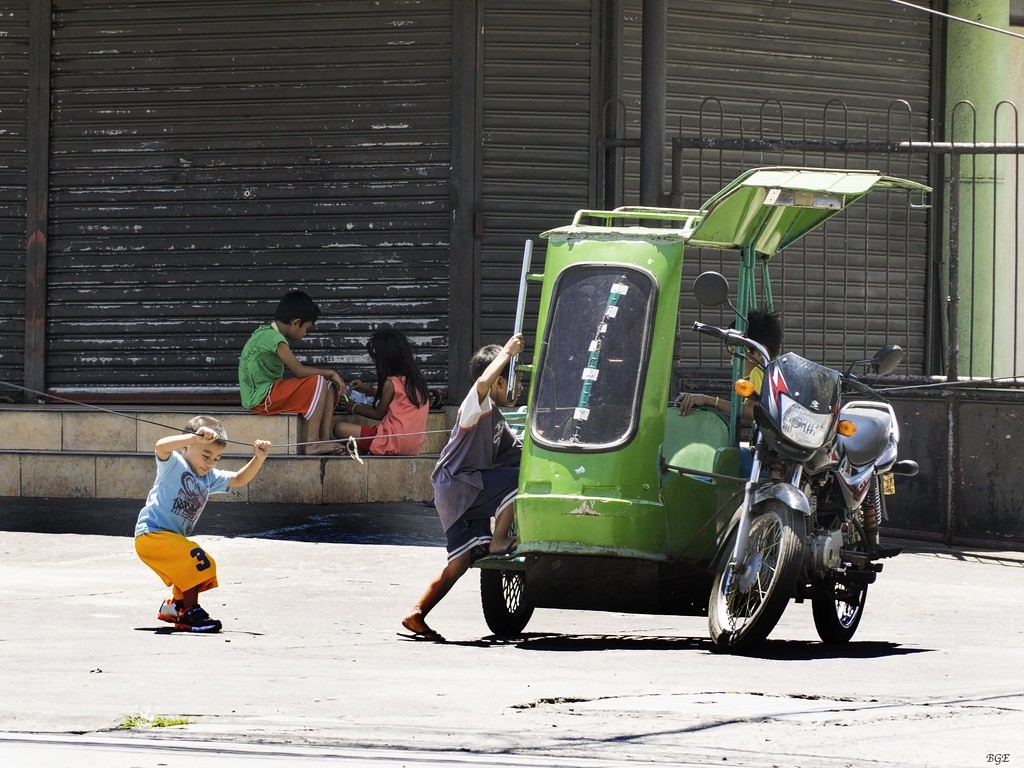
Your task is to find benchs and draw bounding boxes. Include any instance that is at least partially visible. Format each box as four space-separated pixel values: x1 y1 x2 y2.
561 402 731 469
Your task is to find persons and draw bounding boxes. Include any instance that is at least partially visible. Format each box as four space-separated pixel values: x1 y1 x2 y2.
239 289 347 456
134 415 272 634
674 305 783 420
396 333 524 643
332 328 431 456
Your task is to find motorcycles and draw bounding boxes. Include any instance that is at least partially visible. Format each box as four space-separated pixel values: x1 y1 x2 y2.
470 166 933 652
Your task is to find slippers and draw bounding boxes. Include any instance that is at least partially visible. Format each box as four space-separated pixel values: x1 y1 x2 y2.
475 538 518 562
397 628 446 641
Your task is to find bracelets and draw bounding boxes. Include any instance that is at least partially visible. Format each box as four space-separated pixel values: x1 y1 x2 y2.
351 402 360 415
714 396 719 409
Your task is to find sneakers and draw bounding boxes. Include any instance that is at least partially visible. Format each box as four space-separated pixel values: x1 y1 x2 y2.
175 603 222 632
157 599 184 623
